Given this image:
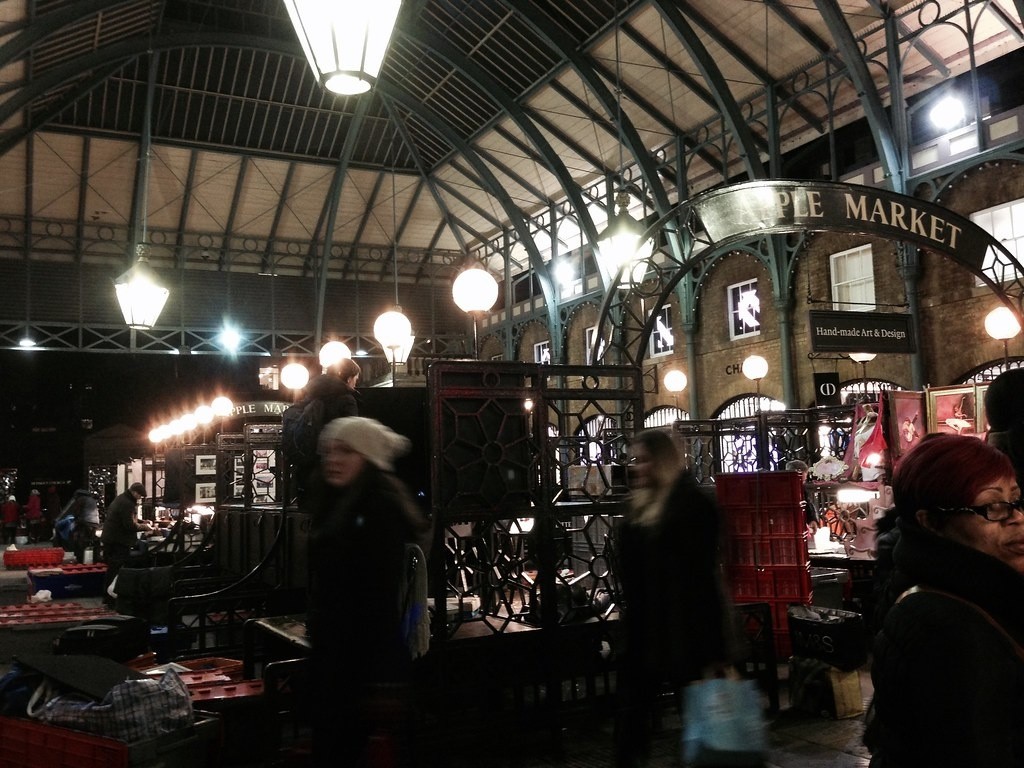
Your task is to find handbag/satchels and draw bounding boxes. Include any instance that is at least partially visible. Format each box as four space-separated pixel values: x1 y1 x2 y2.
788 604 867 719
680 661 768 768
0 625 194 745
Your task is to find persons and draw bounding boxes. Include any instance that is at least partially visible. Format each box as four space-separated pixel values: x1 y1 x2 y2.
102 483 156 606
296 357 361 507
303 416 428 768
785 460 822 538
607 430 735 768
0 484 104 563
860 367 1024 768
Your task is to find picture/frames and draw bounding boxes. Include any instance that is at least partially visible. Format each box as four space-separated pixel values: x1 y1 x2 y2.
889 382 989 457
196 450 275 502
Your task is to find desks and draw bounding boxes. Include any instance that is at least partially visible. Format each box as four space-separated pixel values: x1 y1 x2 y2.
249 615 543 652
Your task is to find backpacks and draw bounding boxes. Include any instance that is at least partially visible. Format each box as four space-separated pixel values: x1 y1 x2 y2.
281 392 349 468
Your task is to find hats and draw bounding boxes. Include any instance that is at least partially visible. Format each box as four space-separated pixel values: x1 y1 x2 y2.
130 482 147 497
8 495 16 502
986 368 1024 431
318 415 411 472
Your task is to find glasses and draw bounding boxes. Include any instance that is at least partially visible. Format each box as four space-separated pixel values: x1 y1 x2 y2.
931 496 1024 522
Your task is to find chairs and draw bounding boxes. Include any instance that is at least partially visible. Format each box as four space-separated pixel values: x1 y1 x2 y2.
118 510 286 653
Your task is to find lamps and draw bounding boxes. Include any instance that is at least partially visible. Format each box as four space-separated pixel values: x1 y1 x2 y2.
113 29 168 328
664 355 768 421
284 0 403 96
280 268 499 390
985 307 1021 371
850 353 878 395
597 13 654 288
148 397 231 443
